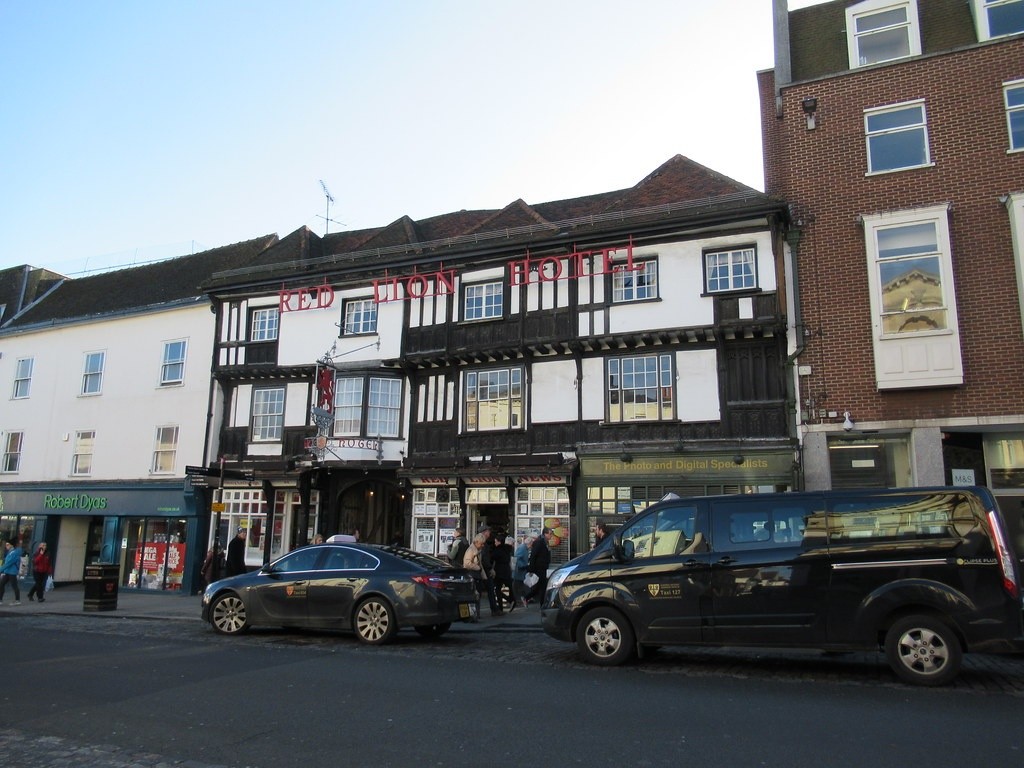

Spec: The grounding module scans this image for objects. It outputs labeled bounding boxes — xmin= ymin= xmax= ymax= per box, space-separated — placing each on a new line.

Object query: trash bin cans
xmin=83 ymin=565 xmax=120 ymax=612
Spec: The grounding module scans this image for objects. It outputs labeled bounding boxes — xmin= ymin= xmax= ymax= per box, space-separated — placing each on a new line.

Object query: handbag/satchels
xmin=524 ymin=572 xmax=540 ymax=587
xmin=45 ymin=574 xmax=54 ymax=593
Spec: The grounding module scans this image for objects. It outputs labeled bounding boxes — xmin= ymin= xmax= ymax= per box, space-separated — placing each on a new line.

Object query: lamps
xmin=801 ymin=98 xmax=817 ymax=130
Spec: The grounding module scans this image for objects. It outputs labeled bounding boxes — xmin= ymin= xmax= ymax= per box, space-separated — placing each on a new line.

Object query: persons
xmin=226 ymin=526 xmax=247 ymax=577
xmin=445 ymin=522 xmax=609 ymax=620
xmin=0 ymin=538 xmax=24 ymax=607
xmin=200 ymin=537 xmax=225 ymax=584
xmin=27 ymin=542 xmax=53 ymax=602
xmin=310 ymin=530 xmax=360 ymax=545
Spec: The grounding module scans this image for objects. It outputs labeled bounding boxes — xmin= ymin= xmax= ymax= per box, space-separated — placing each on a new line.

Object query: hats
xmin=478 ymin=525 xmax=491 ymax=533
xmin=237 ymin=524 xmax=247 ymax=534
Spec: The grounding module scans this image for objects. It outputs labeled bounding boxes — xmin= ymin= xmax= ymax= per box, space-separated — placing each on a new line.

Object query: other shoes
xmin=520 ymin=596 xmax=528 ymax=609
xmin=38 ymin=598 xmax=45 ymax=602
xmin=491 ymin=610 xmax=507 ymax=617
xmin=27 ymin=594 xmax=34 ymax=601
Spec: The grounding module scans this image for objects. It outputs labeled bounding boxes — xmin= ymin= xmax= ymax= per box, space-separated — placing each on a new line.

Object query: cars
xmin=201 ymin=541 xmax=481 ymax=646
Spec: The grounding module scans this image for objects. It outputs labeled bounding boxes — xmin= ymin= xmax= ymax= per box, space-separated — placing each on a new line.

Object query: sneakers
xmin=9 ymin=600 xmax=21 ymax=606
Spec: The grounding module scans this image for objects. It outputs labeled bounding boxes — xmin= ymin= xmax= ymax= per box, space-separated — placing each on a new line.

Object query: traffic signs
xmin=185 ymin=465 xmax=221 ymax=477
xmin=224 ymin=468 xmax=255 ymax=482
xmin=190 ymin=475 xmax=219 ymax=490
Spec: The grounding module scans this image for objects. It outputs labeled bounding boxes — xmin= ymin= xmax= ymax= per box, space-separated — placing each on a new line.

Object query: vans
xmin=542 ymin=485 xmax=1020 ymax=684
